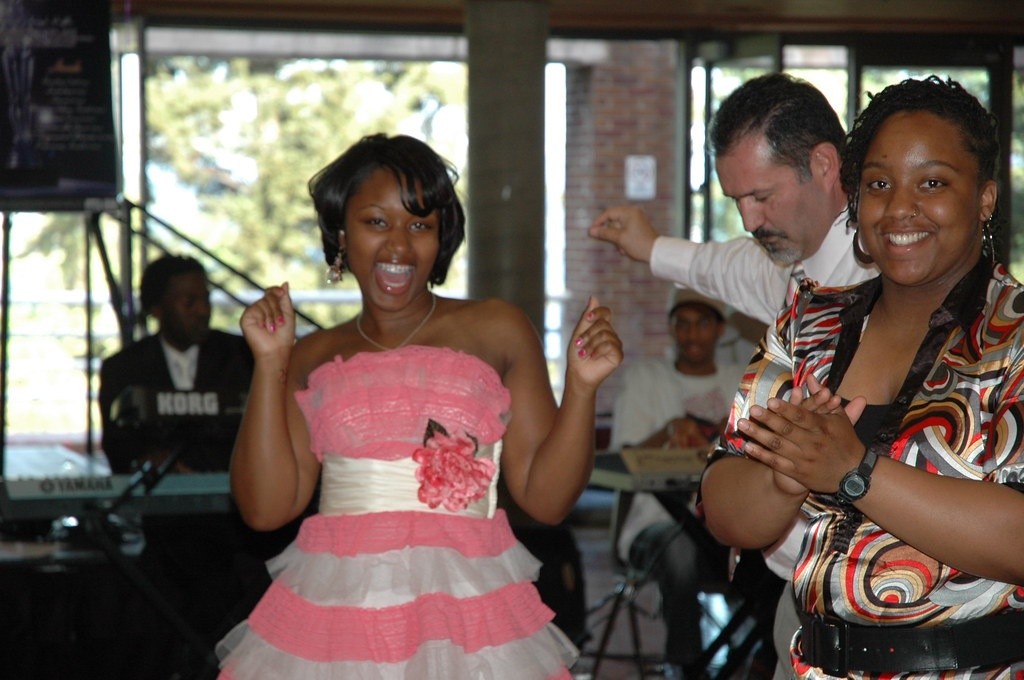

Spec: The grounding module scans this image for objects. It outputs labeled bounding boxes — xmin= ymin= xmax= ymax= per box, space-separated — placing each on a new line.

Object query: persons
xmin=608 ymin=286 xmax=742 ymax=680
xmin=695 ymin=73 xmax=1024 ymax=680
xmin=98 ymin=256 xmax=258 ymax=479
xmin=214 ymin=132 xmax=625 ymax=680
xmin=589 ymin=75 xmax=881 ymax=325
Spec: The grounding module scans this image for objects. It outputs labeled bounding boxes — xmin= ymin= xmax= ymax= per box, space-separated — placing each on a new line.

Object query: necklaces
xmin=356 ymin=292 xmax=436 ymax=352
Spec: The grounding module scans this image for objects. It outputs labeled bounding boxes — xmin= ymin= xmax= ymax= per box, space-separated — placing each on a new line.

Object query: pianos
xmin=0 ymin=468 xmax=232 ymax=529
xmin=586 ymin=448 xmax=708 ymax=493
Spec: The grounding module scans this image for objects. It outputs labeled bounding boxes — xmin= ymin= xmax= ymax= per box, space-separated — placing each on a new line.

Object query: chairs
xmin=608 ymin=495 xmax=679 ymax=680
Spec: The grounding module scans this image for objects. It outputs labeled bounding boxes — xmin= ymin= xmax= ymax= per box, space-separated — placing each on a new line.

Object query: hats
xmin=669 ymin=288 xmax=726 ymax=321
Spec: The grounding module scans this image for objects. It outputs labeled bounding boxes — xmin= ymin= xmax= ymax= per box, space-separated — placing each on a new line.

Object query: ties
xmin=781 ymin=259 xmax=806 ymax=309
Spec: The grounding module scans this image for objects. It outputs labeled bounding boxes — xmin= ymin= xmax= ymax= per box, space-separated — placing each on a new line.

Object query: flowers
xmin=414 ymin=420 xmax=499 ymax=512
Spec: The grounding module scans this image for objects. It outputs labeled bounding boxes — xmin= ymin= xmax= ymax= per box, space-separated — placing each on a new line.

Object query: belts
xmin=799 ymin=612 xmax=1024 ymax=678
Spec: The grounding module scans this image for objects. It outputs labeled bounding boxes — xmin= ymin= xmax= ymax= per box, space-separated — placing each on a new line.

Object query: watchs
xmin=836 ymin=447 xmax=879 ymax=508
